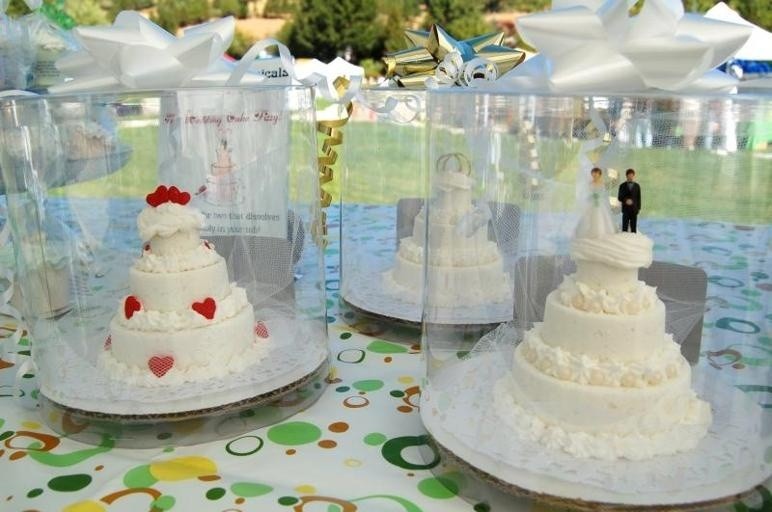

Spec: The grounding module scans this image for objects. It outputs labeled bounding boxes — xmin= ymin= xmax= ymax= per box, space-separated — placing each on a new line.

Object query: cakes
xmin=490 ymin=166 xmax=714 ymax=463
xmin=102 ymin=185 xmax=273 ymax=390
xmin=380 ymin=168 xmax=515 ymax=309
xmin=199 ymin=140 xmax=245 ymax=204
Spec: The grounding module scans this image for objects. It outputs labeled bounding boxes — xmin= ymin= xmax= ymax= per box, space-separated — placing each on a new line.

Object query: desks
xmin=0 ymin=193 xmax=771 ymax=511
